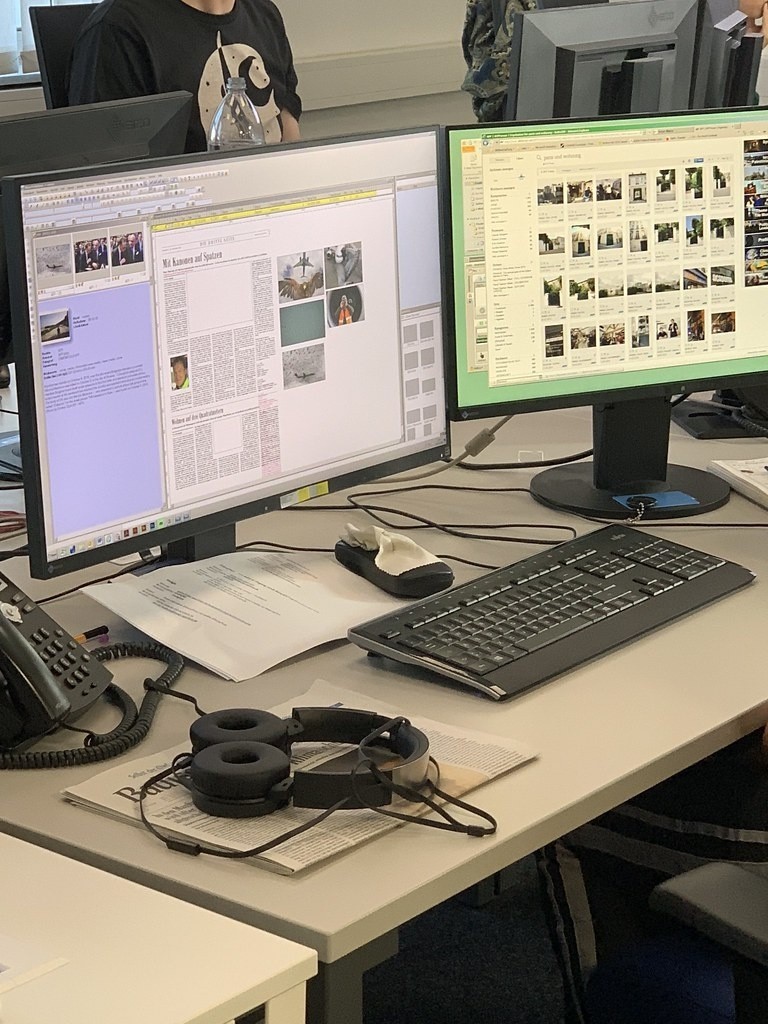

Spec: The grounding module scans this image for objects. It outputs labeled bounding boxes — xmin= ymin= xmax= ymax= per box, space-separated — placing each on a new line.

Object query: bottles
xmin=208 ymin=77 xmax=267 ymax=152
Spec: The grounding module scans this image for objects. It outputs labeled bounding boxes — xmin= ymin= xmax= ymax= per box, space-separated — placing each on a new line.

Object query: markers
xmin=79 ymin=634 xmax=110 ymax=649
xmin=74 ymin=624 xmax=110 ymax=643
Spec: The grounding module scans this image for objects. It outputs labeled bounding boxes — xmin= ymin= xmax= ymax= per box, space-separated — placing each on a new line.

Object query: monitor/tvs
xmin=0 ymin=0 xmax=768 ymax=580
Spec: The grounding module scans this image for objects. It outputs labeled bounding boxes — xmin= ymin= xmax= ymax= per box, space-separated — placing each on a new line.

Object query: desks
xmin=0 ymin=400 xmax=768 ymax=1024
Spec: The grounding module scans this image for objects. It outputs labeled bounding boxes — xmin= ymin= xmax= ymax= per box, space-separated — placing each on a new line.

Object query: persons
xmin=67 ymin=0 xmax=303 ymax=154
xmin=173 ymin=357 xmax=190 ymax=389
xmin=77 ymin=233 xmax=143 ymax=273
xmin=461 ymin=0 xmax=767 ymax=124
xmin=584 ymin=187 xmax=768 ymax=340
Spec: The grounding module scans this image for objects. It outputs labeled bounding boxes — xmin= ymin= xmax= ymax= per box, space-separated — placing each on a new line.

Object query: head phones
xmin=190 ymin=706 xmax=430 ymax=819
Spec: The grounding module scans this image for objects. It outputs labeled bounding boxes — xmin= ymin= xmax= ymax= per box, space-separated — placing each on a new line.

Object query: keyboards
xmin=348 ymin=526 xmax=757 ymax=702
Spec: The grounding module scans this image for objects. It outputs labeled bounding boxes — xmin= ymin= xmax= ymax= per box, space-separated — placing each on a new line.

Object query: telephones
xmin=0 ymin=572 xmax=115 ymax=751
